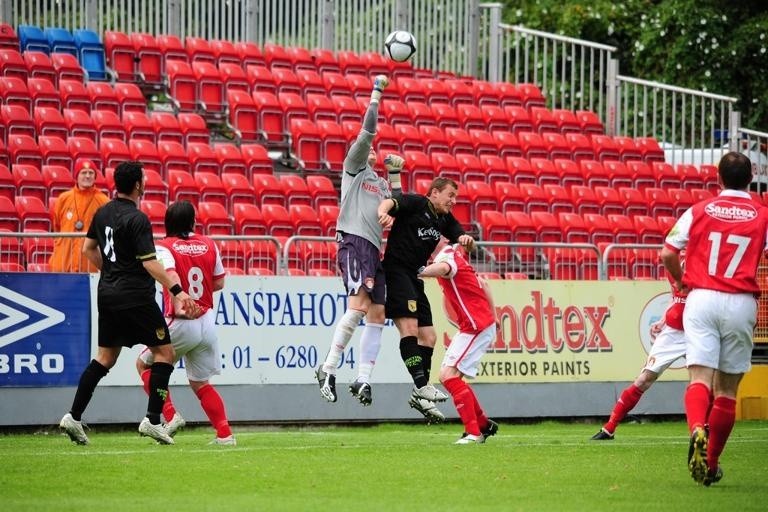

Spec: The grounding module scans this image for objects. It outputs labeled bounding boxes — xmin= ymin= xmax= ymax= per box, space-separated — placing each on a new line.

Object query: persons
xmin=377 ymin=177 xmax=478 ymax=423
xmin=48 ymin=158 xmax=112 ymax=273
xmin=58 ymin=160 xmax=199 ymax=445
xmin=316 ymin=75 xmax=404 ymax=407
xmin=658 ymin=150 xmax=768 ymax=487
xmin=586 ymin=249 xmax=716 ymax=442
xmin=416 ymin=232 xmax=498 ymax=444
xmin=134 ymin=200 xmax=237 ymax=448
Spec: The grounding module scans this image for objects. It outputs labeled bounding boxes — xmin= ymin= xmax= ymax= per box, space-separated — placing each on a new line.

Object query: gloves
xmin=374 ymin=75 xmax=389 ymax=94
xmin=382 ymin=155 xmax=404 ymax=173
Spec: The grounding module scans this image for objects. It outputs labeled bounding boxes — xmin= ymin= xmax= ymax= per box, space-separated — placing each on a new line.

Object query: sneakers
xmin=138 ymin=412 xmax=186 ymax=445
xmin=315 ymin=362 xmax=335 ymax=404
xmin=589 ymin=430 xmax=613 ymax=440
xmin=454 ymin=419 xmax=499 ymax=447
xmin=206 ymin=435 xmax=236 ymax=447
xmin=685 ymin=427 xmax=723 ymax=486
xmin=407 ymin=384 xmax=448 ymax=422
xmin=59 ymin=412 xmax=89 ymax=444
xmin=349 ymin=381 xmax=372 ymax=406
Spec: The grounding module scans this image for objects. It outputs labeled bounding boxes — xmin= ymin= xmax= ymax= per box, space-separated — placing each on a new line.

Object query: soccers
xmin=384 ymin=30 xmax=418 ymax=62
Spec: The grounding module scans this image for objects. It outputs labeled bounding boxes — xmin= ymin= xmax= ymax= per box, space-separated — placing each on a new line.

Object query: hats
xmin=73 ymin=160 xmax=99 ymax=180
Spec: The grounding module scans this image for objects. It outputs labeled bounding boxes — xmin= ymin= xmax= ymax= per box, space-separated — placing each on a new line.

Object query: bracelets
xmin=169 ymin=283 xmax=183 ymax=298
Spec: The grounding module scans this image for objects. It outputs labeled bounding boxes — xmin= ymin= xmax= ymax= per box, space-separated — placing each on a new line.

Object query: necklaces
xmin=72 ymin=188 xmax=97 ymax=230
